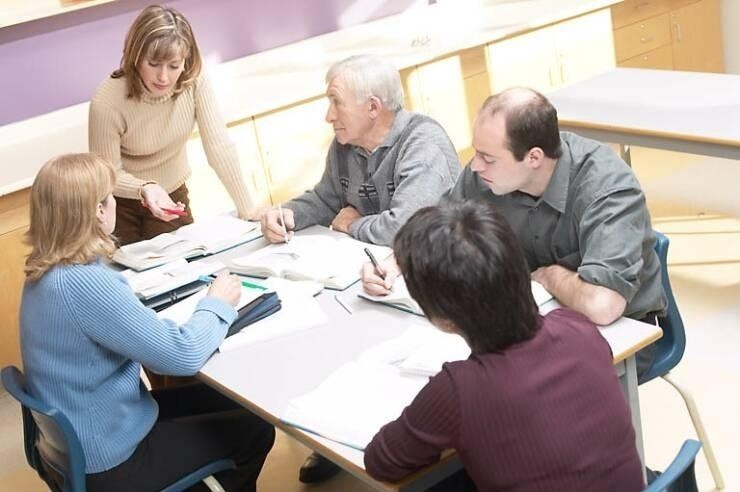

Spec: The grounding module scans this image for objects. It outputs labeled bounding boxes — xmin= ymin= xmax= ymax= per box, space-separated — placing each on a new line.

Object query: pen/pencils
xmin=161 ymin=207 xmax=189 ymax=217
xmin=208 ymin=274 xmax=217 ymax=278
xmin=335 ymin=294 xmax=353 ymax=315
xmin=364 ymin=248 xmax=393 ymax=292
xmin=278 ymin=204 xmax=288 ymax=244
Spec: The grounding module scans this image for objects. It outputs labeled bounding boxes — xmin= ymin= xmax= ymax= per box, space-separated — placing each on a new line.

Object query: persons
xmin=363 ymin=200 xmax=645 ymax=492
xmin=359 ymin=87 xmax=669 ymax=384
xmin=260 ymin=54 xmax=462 ymax=485
xmin=87 ymin=4 xmax=263 ymax=250
xmin=19 ymin=155 xmax=275 ymax=492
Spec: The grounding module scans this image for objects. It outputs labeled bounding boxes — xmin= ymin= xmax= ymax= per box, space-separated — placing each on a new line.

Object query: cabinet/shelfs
xmin=611 ymin=2 xmax=729 ymax=76
xmin=491 ymin=5 xmax=611 ymax=96
xmin=401 ymin=47 xmax=491 ymax=165
xmin=0 ymin=177 xmax=33 ymax=363
xmin=183 ymin=99 xmax=334 ymax=222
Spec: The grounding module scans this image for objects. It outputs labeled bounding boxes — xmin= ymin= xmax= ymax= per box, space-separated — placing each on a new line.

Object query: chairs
xmin=638 ymin=228 xmax=726 ymax=492
xmin=3 ymin=362 xmax=237 ymax=492
xmin=642 ymin=434 xmax=703 ymax=491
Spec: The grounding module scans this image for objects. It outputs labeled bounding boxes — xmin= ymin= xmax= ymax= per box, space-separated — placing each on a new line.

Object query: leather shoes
xmin=299 ymin=450 xmax=341 ymax=481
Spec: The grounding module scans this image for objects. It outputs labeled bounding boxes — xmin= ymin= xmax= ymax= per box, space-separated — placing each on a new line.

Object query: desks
xmin=119 ymin=222 xmax=663 ymax=489
xmin=548 ymin=62 xmax=740 ymax=162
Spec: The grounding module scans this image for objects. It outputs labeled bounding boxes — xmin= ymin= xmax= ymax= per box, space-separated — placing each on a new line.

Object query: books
xmin=358 ymin=254 xmax=554 ymax=318
xmin=112 ymin=215 xmax=375 ymax=338
